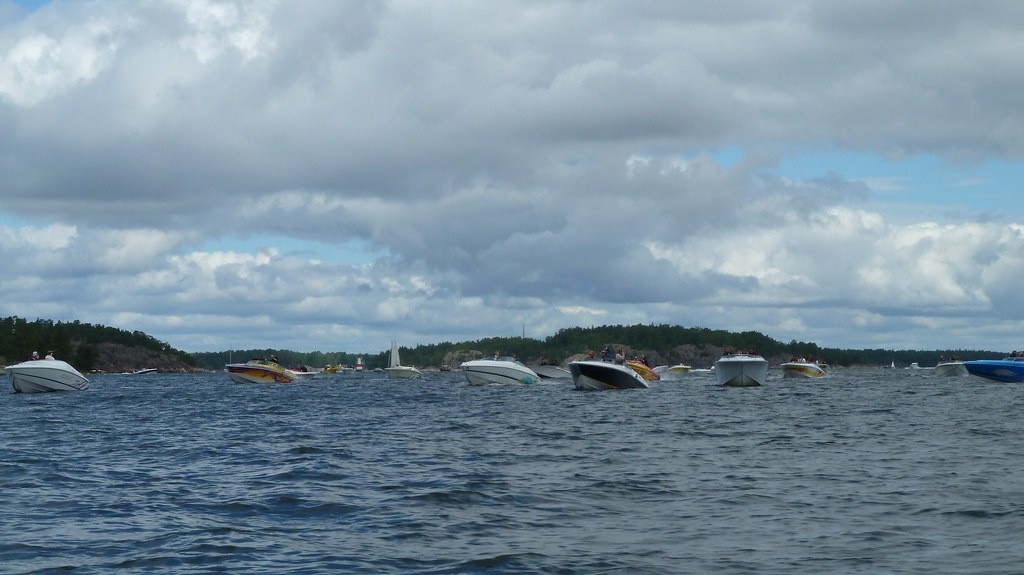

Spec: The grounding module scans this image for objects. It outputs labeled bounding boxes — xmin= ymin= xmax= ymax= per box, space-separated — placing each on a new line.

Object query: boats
xmin=715 ymin=353 xmax=768 ymax=388
xmin=962 ymin=350 xmax=1024 ymax=383
xmin=568 ymin=359 xmax=650 ymax=390
xmin=224 ymin=359 xmax=296 ymax=385
xmin=625 ymin=358 xmax=660 ymax=381
xmin=440 ymin=363 xmax=452 ymax=371
xmin=882 ymin=356 xmax=970 ymax=378
xmin=322 ymin=367 xmax=337 ymax=373
xmin=121 ymin=367 xmax=158 ymax=375
xmin=285 ymin=368 xmax=320 ymax=378
xmin=528 ymin=363 xmax=573 ymax=378
xmin=459 ymin=355 xmax=542 ymax=387
xmin=341 ymin=338 xmax=422 ymax=380
xmin=780 ymin=357 xmax=826 ymax=378
xmin=653 ymin=364 xmax=712 ymax=377
xmin=4 ymin=360 xmax=91 ymax=393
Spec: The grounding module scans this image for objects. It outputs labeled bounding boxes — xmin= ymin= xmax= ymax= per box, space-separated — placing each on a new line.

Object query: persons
xmin=44 ymin=351 xmax=55 ymax=361
xmin=790 ymin=353 xmax=814 ymax=362
xmin=1009 ymin=350 xmax=1017 ymax=357
xmin=722 ymin=345 xmax=757 ymax=356
xmin=585 ymin=345 xmax=625 ymax=365
xmin=270 ymin=354 xmax=280 ymax=365
xmin=29 ymin=351 xmax=40 ymax=361
xmin=492 ymin=350 xmax=517 ymax=362
